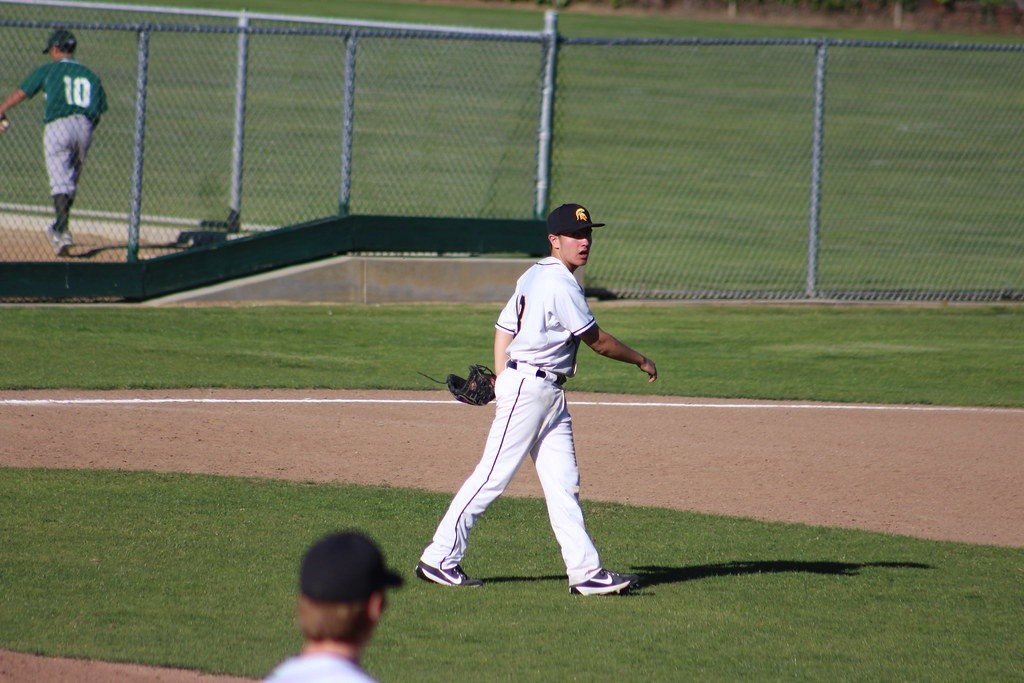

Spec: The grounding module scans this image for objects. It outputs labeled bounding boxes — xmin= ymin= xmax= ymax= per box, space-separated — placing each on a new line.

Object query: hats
xmin=300 ymin=533 xmax=404 ymax=601
xmin=547 ymin=203 xmax=605 ymax=234
xmin=43 ymin=29 xmax=76 ymax=54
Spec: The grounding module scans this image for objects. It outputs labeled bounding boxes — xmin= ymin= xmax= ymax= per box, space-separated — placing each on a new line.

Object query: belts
xmin=507 ymin=360 xmax=566 ymax=385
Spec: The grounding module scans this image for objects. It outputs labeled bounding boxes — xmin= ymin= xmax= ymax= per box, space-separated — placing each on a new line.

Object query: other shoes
xmin=48 ymin=225 xmax=73 ymax=255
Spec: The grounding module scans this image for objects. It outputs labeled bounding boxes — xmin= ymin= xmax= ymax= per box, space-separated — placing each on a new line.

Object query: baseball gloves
xmin=447 ymin=364 xmax=496 ymax=405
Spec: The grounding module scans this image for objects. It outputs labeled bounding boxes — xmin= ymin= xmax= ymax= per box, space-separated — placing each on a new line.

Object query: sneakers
xmin=415 ymin=560 xmax=483 ymax=588
xmin=569 ymin=569 xmax=640 ymax=596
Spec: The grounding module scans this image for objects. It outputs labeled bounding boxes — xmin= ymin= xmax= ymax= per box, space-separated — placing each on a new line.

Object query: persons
xmin=261 ymin=530 xmax=403 ymax=683
xmin=415 ymin=204 xmax=658 ymax=596
xmin=0 ymin=31 xmax=108 ymax=256
